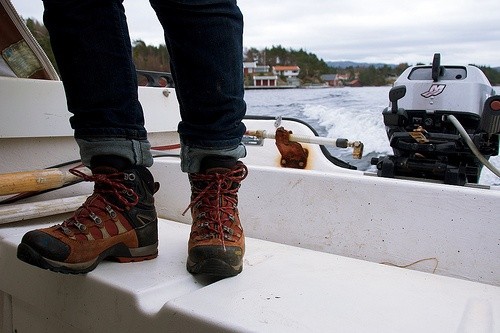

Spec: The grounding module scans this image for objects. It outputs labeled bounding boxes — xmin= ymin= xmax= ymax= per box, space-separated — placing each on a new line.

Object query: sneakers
xmin=17 ymin=154 xmax=160 ymax=275
xmin=182 ymin=154 xmax=248 ymax=275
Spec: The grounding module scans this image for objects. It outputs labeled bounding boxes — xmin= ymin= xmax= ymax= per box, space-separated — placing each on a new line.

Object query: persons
xmin=17 ymin=0 xmax=248 ymax=277
